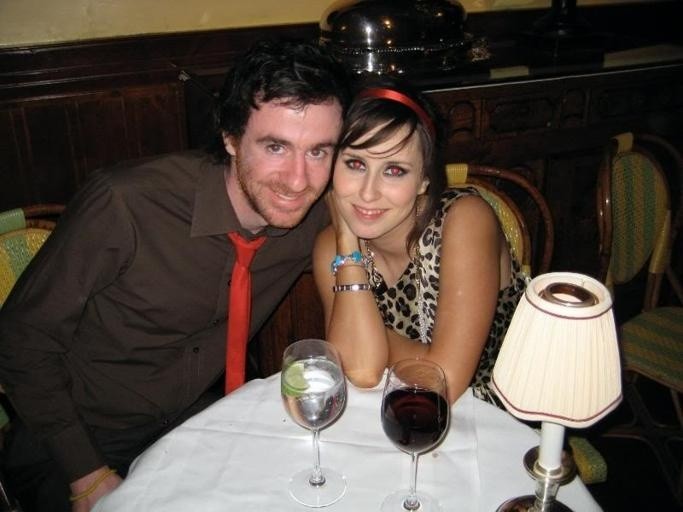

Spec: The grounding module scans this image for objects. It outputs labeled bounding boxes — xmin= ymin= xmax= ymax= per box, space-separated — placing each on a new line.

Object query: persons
xmin=312 ymin=84 xmax=532 ymax=412
xmin=0 ymin=44 xmax=354 ymax=512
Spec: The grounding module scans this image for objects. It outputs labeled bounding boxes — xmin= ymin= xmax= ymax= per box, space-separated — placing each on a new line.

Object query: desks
xmin=90 ymin=356 xmax=604 ymax=512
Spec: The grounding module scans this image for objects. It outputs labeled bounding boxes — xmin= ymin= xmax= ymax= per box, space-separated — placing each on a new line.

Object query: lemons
xmin=281 ymin=361 xmax=311 ymax=394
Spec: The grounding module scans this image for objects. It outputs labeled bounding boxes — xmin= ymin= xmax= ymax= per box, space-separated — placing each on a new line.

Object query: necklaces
xmin=365 ymin=202 xmax=427 ymax=344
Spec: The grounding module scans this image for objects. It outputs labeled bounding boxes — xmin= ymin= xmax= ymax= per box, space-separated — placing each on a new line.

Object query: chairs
xmin=0 ymin=202 xmax=81 ymax=512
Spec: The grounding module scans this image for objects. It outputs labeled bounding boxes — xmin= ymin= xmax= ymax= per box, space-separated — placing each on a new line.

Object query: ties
xmin=225 ymin=231 xmax=267 ymax=393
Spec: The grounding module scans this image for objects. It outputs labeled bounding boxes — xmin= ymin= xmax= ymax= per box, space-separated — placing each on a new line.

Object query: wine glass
xmin=280 ymin=338 xmax=347 ymax=508
xmin=379 ymin=358 xmax=448 ymax=512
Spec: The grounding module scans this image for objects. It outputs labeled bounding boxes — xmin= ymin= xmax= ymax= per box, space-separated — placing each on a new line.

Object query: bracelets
xmin=332 ymin=284 xmax=371 ymax=291
xmin=70 ymin=469 xmax=117 ymax=502
xmin=332 ymin=251 xmax=364 ymax=276
